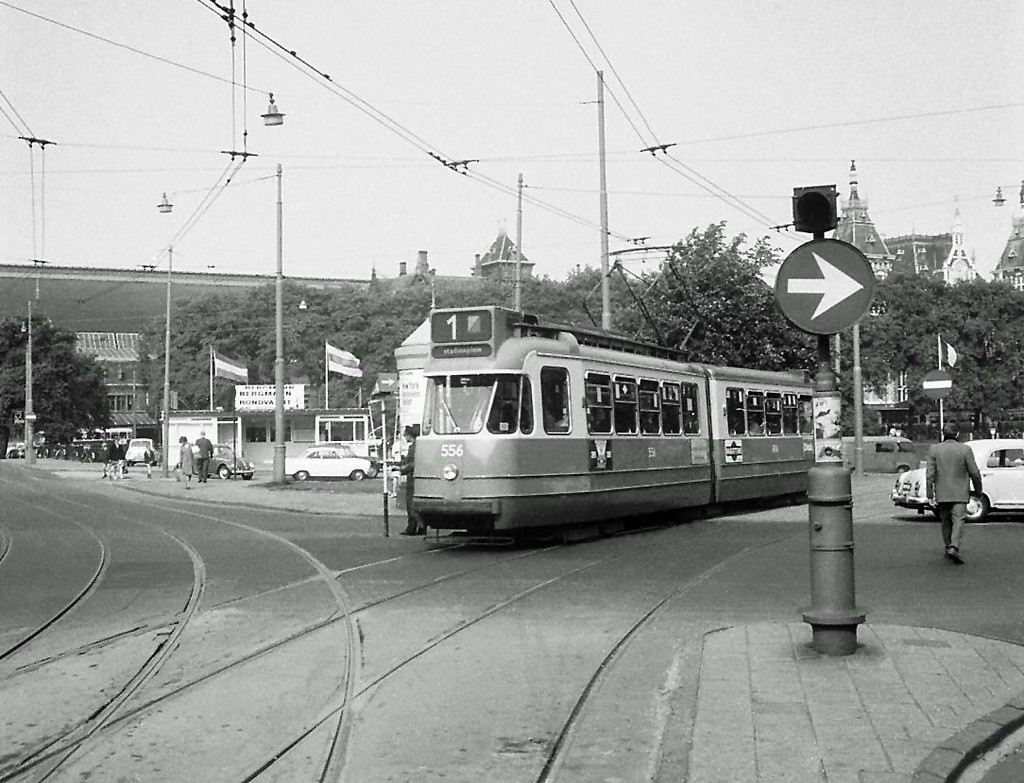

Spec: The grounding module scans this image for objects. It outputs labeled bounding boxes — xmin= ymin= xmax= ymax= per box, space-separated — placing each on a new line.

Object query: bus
xmin=409 ymin=245 xmax=815 ymax=549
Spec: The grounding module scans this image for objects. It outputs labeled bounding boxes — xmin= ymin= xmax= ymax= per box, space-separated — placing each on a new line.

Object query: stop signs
xmin=921 ymin=370 xmax=954 ymax=400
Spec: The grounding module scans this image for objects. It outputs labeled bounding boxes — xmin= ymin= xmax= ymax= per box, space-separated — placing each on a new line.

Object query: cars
xmin=124 ymin=437 xmax=162 ymax=467
xmin=173 ymin=442 xmax=256 ymax=481
xmin=285 ymin=443 xmax=373 ymax=482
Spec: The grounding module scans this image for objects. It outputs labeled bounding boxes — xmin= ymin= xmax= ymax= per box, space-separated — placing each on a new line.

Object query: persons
xmin=196 ymin=432 xmax=215 ymax=483
xmin=925 ymin=423 xmax=982 ymax=564
xmin=101 ymin=436 xmax=156 ymax=480
xmin=178 ymin=436 xmax=197 ymax=489
xmin=390 ymin=426 xmax=428 ymax=536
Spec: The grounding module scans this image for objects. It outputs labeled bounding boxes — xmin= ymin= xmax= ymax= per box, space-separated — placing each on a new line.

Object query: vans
xmin=888 ymin=439 xmax=1024 ymax=523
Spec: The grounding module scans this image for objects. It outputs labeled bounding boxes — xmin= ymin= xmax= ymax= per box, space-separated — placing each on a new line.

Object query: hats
xmin=179 ymin=436 xmax=187 ymax=443
xmin=402 ymin=425 xmax=419 ymax=435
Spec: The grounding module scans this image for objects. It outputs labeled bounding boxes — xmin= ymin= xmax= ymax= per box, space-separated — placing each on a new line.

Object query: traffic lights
xmin=792 ymin=184 xmax=838 ymax=233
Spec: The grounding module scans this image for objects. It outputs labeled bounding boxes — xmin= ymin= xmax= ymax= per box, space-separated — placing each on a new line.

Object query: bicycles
xmin=7 ymin=436 xmax=95 ymax=463
xmin=107 ymin=460 xmax=125 ymax=481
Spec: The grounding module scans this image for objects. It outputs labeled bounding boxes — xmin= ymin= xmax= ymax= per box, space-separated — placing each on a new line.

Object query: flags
xmin=940 ymin=340 xmax=961 ymax=372
xmin=213 ymin=348 xmax=248 ymax=384
xmin=327 ymin=344 xmax=363 ymax=377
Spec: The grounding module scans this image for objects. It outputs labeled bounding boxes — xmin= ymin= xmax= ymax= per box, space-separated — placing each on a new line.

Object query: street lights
xmin=19 ymin=300 xmax=55 ymax=464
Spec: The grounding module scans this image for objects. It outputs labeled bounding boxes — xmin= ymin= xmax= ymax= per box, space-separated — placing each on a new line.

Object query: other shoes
xmin=399 ymin=528 xmax=417 ymax=535
xmin=101 ymin=474 xmax=108 ymax=479
xmin=417 ymin=526 xmax=427 ymax=535
xmin=943 ymin=548 xmax=964 ymax=564
